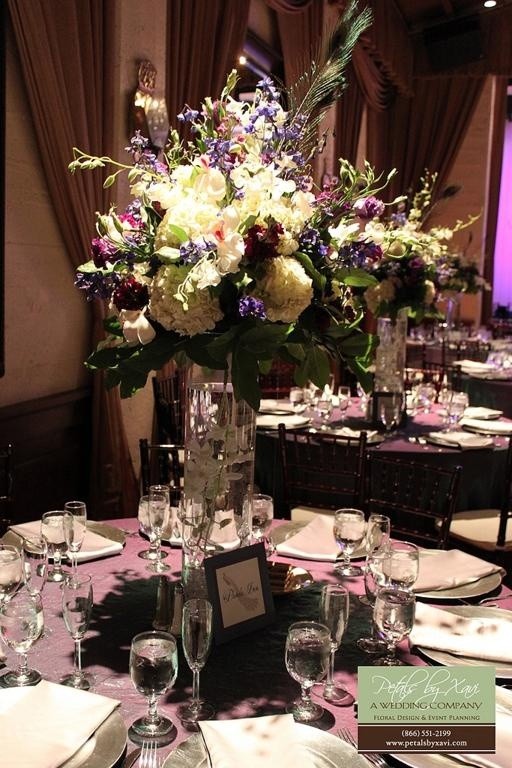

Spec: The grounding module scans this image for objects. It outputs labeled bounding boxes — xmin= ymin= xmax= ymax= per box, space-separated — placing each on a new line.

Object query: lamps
xmin=127 ymin=60 xmax=171 ymax=162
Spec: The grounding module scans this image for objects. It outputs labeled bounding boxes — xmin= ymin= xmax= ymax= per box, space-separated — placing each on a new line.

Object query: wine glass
xmin=0 ymin=590 xmax=46 ymax=689
xmin=136 ymin=482 xmax=177 ymax=573
xmin=287 ymin=373 xmax=355 ymax=431
xmin=127 ymin=631 xmax=180 ymax=747
xmin=282 ymin=620 xmax=334 ymax=723
xmin=1 ymin=501 xmax=88 ymax=602
xmin=175 ymin=597 xmax=217 ymax=724
xmin=312 ymin=582 xmax=352 ymax=700
xmin=332 ymin=505 xmax=418 ymax=672
xmin=58 ymin=573 xmax=96 ymax=690
xmin=246 ymin=493 xmax=275 ymax=558
xmin=415 ymin=382 xmax=466 ymax=435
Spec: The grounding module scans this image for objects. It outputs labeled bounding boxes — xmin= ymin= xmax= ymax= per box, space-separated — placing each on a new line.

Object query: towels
xmin=1 ymin=680 xmax=122 ymax=767
xmin=197 ymin=713 xmax=314 ymax=768
xmin=1 ymin=516 xmax=512 ymax=766
xmin=368 ymin=546 xmax=504 ymax=600
xmin=404 ymin=601 xmax=511 ymax=663
xmin=275 ymin=516 xmax=375 ymax=561
xmin=9 ymin=514 xmax=122 ymax=568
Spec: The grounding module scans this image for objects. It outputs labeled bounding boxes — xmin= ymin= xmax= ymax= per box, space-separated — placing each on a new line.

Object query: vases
xmin=181 ymin=348 xmax=257 ymax=600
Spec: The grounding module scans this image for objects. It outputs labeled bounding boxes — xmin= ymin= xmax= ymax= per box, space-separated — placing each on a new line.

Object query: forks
xmin=336 ymin=725 xmax=390 ymax=767
xmin=138 ymin=740 xmax=159 ymax=768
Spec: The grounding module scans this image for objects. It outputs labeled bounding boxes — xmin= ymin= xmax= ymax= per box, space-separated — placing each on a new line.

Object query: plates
xmin=373 ymin=547 xmax=503 ymax=601
xmin=254 ymin=398 xmax=386 ymax=447
xmin=161 ymin=712 xmax=373 ymax=767
xmin=417 ymin=404 xmax=511 ymax=449
xmin=9 ymin=516 xmax=127 ymax=564
xmin=58 ymin=698 xmax=130 ymax=768
xmin=265 ymin=515 xmax=383 ymax=562
xmin=411 ymin=604 xmax=512 ymax=680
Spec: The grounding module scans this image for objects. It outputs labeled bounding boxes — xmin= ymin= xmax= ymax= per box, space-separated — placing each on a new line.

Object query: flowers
xmin=68 ymin=0 xmax=484 ymax=414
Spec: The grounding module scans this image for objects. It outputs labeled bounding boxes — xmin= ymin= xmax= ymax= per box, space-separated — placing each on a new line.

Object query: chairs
xmin=139 ymin=336 xmax=512 ymax=550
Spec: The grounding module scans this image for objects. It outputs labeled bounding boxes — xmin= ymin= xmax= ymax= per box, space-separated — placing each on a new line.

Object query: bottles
xmin=178 ymin=345 xmax=263 ymax=608
